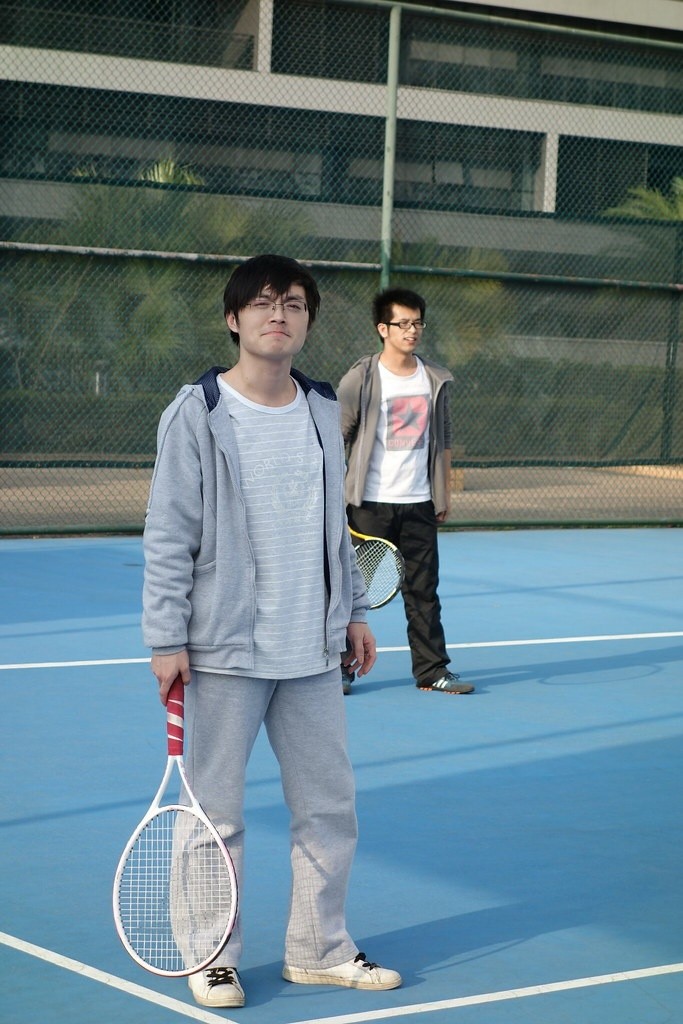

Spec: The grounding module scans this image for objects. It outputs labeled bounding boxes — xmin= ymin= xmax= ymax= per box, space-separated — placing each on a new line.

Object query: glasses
xmin=245 ymin=300 xmax=308 ymax=315
xmin=385 ymin=319 xmax=427 ymax=329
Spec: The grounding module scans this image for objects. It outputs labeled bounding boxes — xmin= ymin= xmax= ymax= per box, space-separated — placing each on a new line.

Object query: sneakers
xmin=342 ymin=673 xmax=351 ymax=694
xmin=282 ymin=952 xmax=402 ymax=990
xmin=188 ymin=965 xmax=245 ymax=1006
xmin=420 ymin=671 xmax=475 ymax=694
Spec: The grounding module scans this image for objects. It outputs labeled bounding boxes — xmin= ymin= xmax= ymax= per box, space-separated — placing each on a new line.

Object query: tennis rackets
xmin=112 ymin=677 xmax=240 ymax=979
xmin=346 ymin=526 xmax=407 ymax=610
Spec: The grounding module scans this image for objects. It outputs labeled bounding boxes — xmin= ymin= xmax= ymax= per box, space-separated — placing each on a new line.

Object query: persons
xmin=141 ymin=254 xmax=403 ymax=1008
xmin=333 ymin=290 xmax=475 ymax=695
